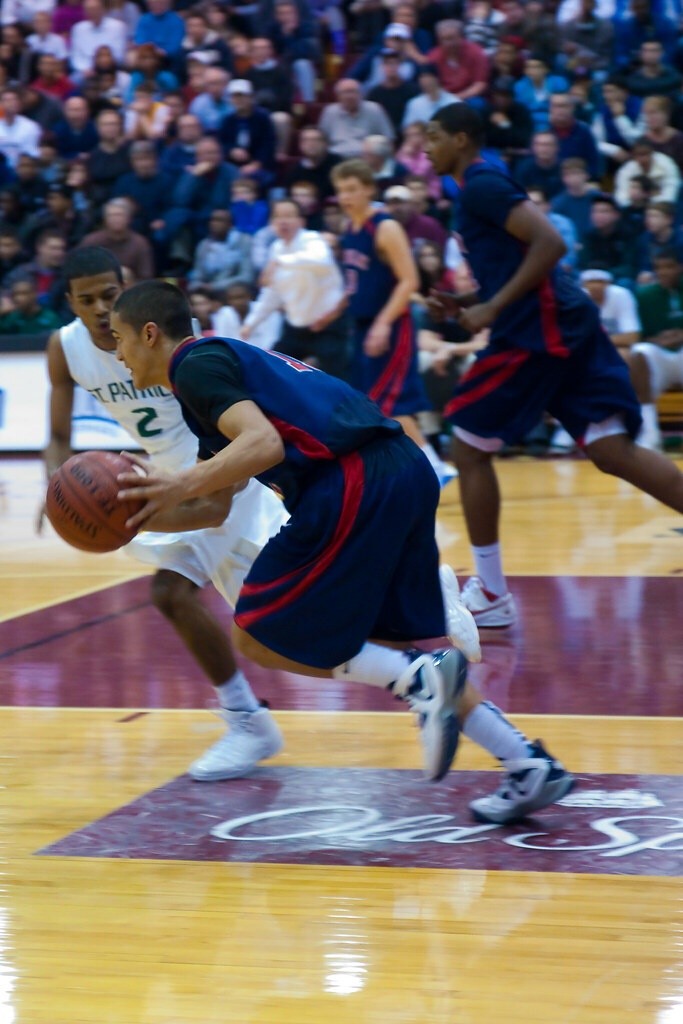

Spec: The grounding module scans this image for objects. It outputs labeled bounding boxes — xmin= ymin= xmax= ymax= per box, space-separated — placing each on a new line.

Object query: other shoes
xmin=497 ymin=438 xmax=548 ymax=459
xmin=549 ymin=426 xmax=575 ymax=454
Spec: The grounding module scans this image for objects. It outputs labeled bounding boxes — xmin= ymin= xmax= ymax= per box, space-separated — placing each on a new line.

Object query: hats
xmin=226 ymin=79 xmax=251 ymax=95
xmin=386 ymin=22 xmax=411 ymax=39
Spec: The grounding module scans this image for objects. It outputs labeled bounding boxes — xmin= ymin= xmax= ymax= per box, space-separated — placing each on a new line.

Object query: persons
xmin=36 ymin=244 xmax=469 ymax=783
xmin=0 ymin=1 xmax=683 ymax=451
xmin=109 ymin=280 xmax=577 ymax=826
xmin=330 ymin=159 xmax=459 ymax=488
xmin=422 ymin=102 xmax=683 ymax=630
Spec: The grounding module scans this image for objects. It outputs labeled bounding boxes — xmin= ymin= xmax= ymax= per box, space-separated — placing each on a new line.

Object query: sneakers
xmin=384 ymin=645 xmax=468 ymax=783
xmin=469 ymin=738 xmax=574 ymax=823
xmin=191 ymin=698 xmax=283 ymax=780
xmin=459 ymin=577 xmax=516 ymax=626
xmin=439 ymin=564 xmax=481 ymax=663
xmin=437 ymin=462 xmax=458 ymax=489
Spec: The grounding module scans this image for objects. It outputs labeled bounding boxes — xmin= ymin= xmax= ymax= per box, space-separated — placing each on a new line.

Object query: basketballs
xmin=45 ymin=451 xmax=149 ymax=553
